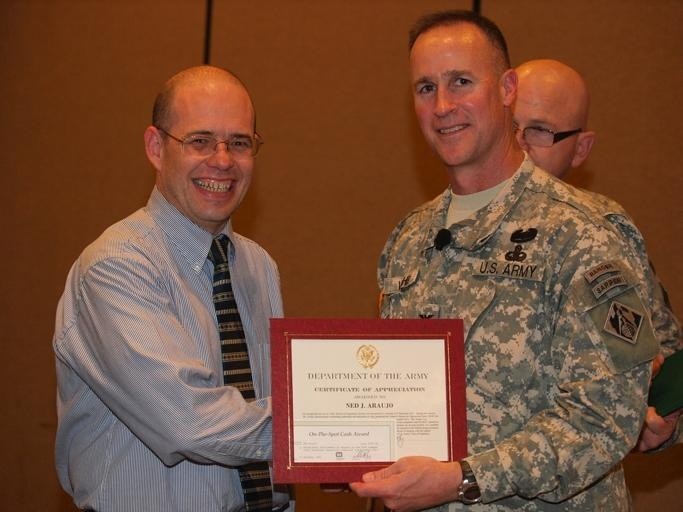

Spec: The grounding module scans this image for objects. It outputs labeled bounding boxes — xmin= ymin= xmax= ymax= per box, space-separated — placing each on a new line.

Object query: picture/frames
xmin=270 ymin=317 xmax=467 ymax=484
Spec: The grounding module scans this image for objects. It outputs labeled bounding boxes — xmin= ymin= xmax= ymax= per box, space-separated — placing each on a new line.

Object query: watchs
xmin=457 ymin=459 xmax=482 ymax=505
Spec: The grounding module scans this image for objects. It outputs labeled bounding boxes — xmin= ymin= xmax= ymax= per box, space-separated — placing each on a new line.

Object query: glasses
xmin=155 ymin=126 xmax=262 ymax=157
xmin=515 ymin=124 xmax=583 ymax=149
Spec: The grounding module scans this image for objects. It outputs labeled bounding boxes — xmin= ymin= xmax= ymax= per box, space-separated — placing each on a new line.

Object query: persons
xmin=505 ymin=59 xmax=683 ymax=456
xmin=49 ymin=64 xmax=300 ymax=511
xmin=349 ymin=9 xmax=661 ymax=512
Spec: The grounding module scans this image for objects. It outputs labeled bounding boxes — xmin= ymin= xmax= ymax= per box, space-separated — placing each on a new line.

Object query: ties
xmin=207 ymin=235 xmax=272 ymax=510
